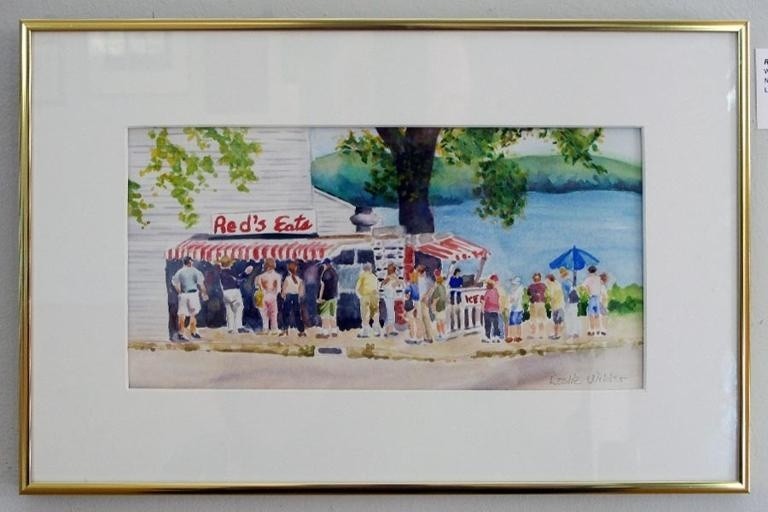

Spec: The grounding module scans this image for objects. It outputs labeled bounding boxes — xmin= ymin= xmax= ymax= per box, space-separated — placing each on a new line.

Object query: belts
xmin=180 ymin=289 xmax=198 ymax=294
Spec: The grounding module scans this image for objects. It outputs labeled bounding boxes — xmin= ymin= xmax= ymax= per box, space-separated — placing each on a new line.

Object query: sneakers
xmin=527 ymin=333 xmax=579 ymax=340
xmin=238 ymin=327 xmax=250 ymax=334
xmin=481 ymin=336 xmax=523 ymax=344
xmin=587 ymin=330 xmax=607 ymax=337
xmin=404 ymin=332 xmax=447 ymax=345
xmin=190 ymin=332 xmax=201 ymax=339
xmin=357 ymin=331 xmax=399 ymax=338
xmin=332 ymin=333 xmax=338 ymax=337
xmin=256 ymin=328 xmax=307 ymax=337
xmin=177 ymin=334 xmax=189 ymax=342
xmin=316 ymin=333 xmax=330 ymax=339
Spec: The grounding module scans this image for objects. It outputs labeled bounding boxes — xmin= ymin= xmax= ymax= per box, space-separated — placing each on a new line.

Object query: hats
xmin=321 ymin=258 xmax=331 ymax=265
xmin=220 ymin=255 xmax=234 ymax=268
xmin=182 ymin=256 xmax=195 ymax=265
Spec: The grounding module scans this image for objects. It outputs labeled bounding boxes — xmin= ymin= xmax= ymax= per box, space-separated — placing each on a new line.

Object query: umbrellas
xmin=549 ymin=244 xmax=602 ymax=285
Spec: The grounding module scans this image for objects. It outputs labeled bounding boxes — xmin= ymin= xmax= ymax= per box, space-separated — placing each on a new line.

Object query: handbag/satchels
xmin=252 ymin=277 xmax=264 ymax=308
xmin=404 ymin=285 xmax=414 ymax=312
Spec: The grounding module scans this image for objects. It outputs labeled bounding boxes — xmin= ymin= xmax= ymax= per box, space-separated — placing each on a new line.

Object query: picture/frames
xmin=17 ymin=17 xmax=750 ymax=494
